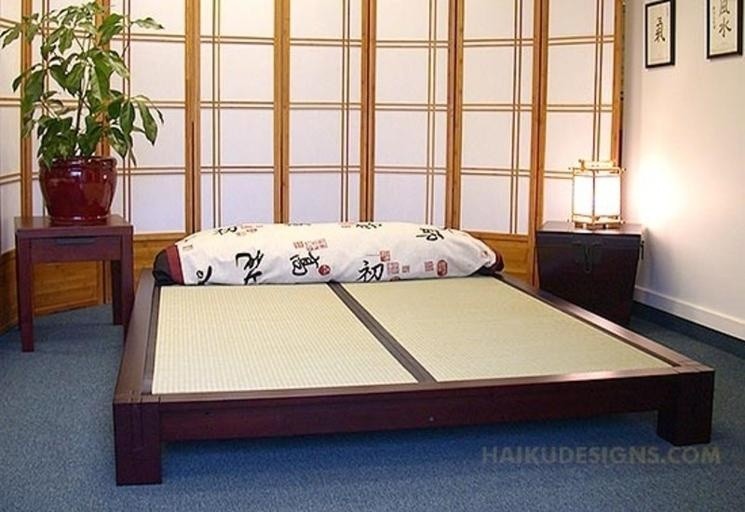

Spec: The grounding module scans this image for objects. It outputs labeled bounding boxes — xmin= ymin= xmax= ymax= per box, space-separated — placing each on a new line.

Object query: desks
xmin=13 ymin=213 xmax=136 ymax=351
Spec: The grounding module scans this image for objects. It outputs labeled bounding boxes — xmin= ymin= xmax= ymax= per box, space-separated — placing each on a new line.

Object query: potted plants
xmin=0 ymin=0 xmax=167 ymax=223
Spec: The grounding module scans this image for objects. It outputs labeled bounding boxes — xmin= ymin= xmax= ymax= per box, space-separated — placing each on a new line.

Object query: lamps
xmin=570 ymin=158 xmax=630 ymax=230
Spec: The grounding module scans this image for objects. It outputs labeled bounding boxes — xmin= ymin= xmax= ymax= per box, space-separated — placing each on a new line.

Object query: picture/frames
xmin=707 ymin=0 xmax=743 ymax=58
xmin=644 ymin=2 xmax=676 ymax=68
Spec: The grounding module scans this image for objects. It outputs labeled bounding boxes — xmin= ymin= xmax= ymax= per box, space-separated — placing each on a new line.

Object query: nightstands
xmin=535 ymin=221 xmax=644 ymax=328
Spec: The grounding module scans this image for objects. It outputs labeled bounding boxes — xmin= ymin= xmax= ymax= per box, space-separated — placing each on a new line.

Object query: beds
xmin=112 ymin=221 xmax=716 ymax=484
